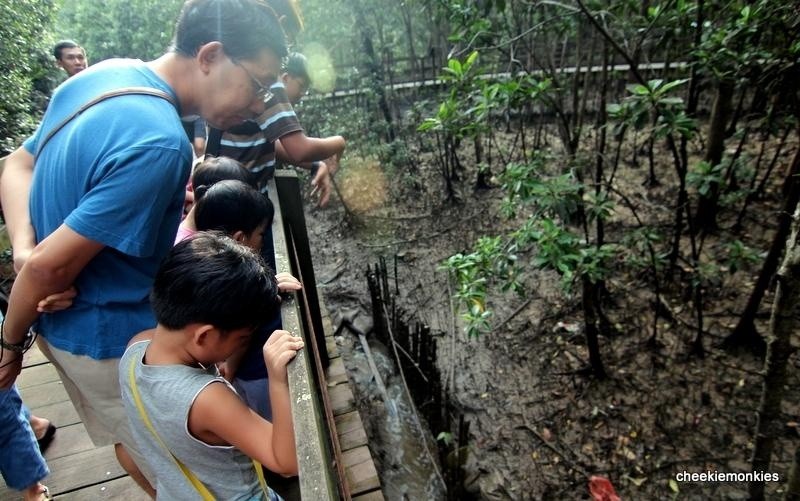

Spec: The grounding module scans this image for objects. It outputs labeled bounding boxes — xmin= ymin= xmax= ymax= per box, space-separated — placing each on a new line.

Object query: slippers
xmin=36 ymin=423 xmax=57 ymax=453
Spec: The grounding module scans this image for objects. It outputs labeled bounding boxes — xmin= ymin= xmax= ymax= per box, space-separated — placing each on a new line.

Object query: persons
xmin=0 ymin=0 xmax=347 ymax=501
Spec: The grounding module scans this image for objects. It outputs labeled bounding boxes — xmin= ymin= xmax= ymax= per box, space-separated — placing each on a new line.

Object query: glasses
xmin=238 ymin=61 xmax=275 ymax=104
